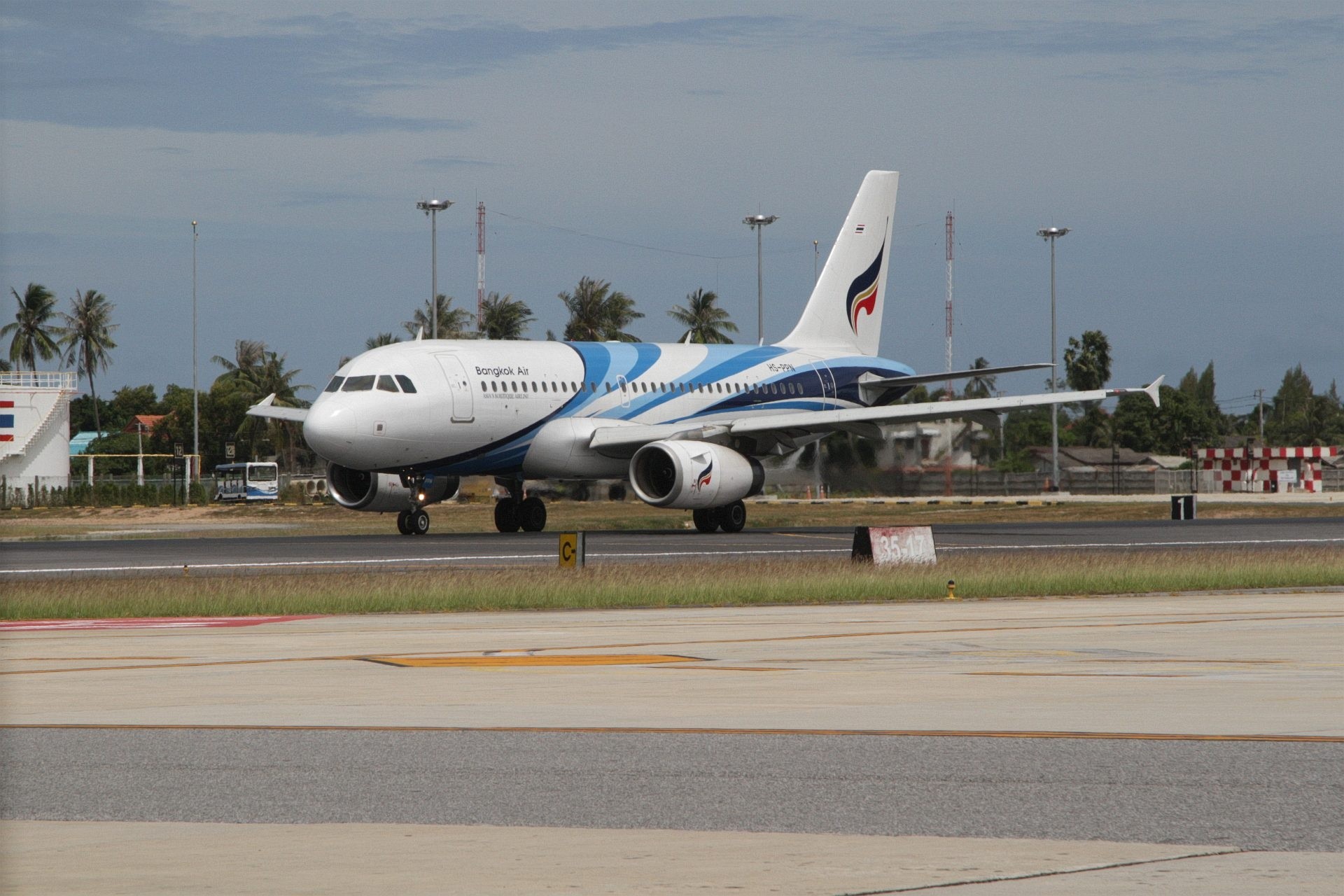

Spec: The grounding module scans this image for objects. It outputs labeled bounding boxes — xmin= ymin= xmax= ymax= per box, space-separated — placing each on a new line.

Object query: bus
xmin=212 ymin=461 xmax=279 ymax=504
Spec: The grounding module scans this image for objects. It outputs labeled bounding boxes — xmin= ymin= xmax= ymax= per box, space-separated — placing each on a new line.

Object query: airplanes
xmin=243 ymin=171 xmax=1171 ymax=535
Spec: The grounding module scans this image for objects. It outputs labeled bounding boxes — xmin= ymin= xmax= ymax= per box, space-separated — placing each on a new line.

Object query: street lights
xmin=415 ymin=196 xmax=456 ymax=340
xmin=1033 ymin=225 xmax=1075 ymax=492
xmin=740 ymin=213 xmax=780 ymax=343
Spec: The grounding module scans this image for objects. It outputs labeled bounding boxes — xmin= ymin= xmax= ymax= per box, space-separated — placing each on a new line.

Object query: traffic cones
xmin=806 ymin=484 xmax=812 ymax=500
xmin=820 ymin=484 xmax=826 ymax=500
